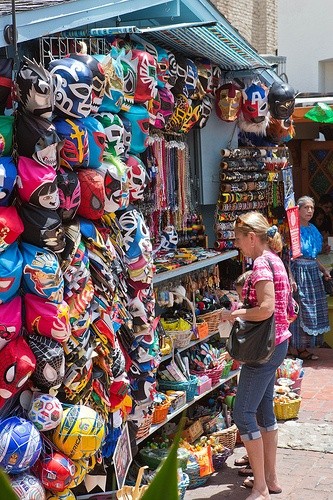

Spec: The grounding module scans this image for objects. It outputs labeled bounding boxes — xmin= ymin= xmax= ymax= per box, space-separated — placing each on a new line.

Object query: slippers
xmin=242 ymin=478 xmax=282 ymax=494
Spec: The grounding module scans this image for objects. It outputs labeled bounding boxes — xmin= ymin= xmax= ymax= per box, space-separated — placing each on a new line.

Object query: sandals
xmin=234 ymin=455 xmax=249 ymax=466
xmin=286 ymin=353 xmax=296 ymax=360
xmin=298 ymin=350 xmax=319 ymax=360
xmin=238 ymin=465 xmax=254 ymax=477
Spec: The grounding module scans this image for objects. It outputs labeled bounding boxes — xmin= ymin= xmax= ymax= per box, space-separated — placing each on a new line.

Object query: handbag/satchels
xmin=225 ymin=258 xmax=276 ymax=364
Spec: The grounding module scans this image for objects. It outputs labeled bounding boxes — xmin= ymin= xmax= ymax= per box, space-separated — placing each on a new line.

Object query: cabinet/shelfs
xmin=129 ymin=250 xmax=240 ymax=444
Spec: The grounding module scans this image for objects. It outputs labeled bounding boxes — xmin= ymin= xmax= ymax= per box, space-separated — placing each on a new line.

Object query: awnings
xmin=0 ymin=0 xmax=283 ymax=87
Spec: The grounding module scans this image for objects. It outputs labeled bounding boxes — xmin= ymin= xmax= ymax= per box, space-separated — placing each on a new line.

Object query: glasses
xmin=236 ymin=217 xmax=254 ymax=231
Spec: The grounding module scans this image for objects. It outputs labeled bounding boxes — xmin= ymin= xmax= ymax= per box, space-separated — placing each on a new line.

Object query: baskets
xmin=136 ymin=358 xmax=240 ymax=440
xmin=159 ymin=292 xmax=196 ymax=347
xmin=208 ymin=423 xmax=238 ymax=450
xmin=138 ymin=448 xmax=210 ymax=489
xmin=273 ymin=396 xmax=302 ymax=420
xmin=141 ymin=471 xmax=190 ymax=500
xmin=194 ymin=306 xmax=225 ymax=332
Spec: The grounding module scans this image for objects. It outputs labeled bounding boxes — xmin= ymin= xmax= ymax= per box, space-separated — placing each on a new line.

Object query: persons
xmin=232 ymin=211 xmax=296 ymax=500
xmin=284 ymin=196 xmax=333 ymax=359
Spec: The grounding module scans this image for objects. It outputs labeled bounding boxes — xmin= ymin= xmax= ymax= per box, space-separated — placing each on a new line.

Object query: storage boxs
xmin=169 ymin=420 xmax=203 ymax=444
